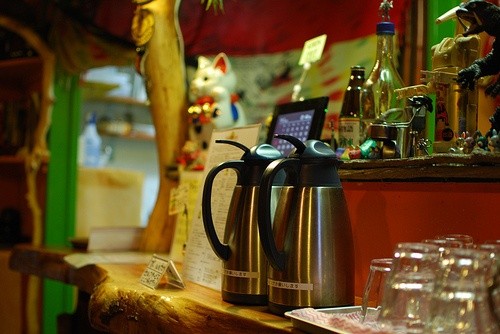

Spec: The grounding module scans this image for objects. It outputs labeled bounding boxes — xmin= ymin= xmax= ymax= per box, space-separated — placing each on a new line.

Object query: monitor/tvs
xmin=265 ymin=95 xmax=330 ymax=158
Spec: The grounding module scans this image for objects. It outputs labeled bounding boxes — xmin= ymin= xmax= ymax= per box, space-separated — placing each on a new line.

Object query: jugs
xmin=260 ymin=134 xmax=350 ymax=317
xmin=203 ymin=139 xmax=288 ymax=306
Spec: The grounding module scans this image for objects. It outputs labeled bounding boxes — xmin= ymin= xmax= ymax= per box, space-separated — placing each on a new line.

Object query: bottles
xmin=338 ymin=65 xmax=367 ymax=161
xmin=429 ymin=34 xmax=479 ymax=151
xmin=75 ymin=112 xmax=101 ymax=170
xmin=359 ymin=22 xmax=406 ymax=159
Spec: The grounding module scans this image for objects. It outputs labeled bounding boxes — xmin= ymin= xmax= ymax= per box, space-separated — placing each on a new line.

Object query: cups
xmin=362 ymin=234 xmax=500 ymax=333
xmin=369 ymin=121 xmax=415 ymax=159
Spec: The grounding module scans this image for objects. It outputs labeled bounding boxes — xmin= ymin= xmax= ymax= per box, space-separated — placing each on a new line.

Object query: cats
xmin=186 ymin=51 xmax=246 ymax=172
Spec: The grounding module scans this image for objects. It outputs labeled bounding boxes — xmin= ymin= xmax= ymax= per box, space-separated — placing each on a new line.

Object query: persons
xmin=79 ymin=111 xmax=152 ymax=166
